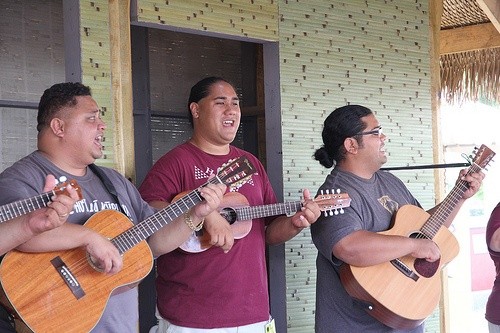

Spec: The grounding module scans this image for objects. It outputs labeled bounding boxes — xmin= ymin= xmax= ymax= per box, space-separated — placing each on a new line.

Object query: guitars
xmin=171 ymin=189 xmax=352 ymax=254
xmin=0 ymin=176 xmax=84 ymax=223
xmin=0 ymin=156 xmax=257 ymax=332
xmin=340 ymin=143 xmax=496 ymax=330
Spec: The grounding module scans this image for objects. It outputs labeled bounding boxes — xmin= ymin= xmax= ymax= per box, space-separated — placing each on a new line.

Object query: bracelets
xmin=185 ymin=205 xmax=207 ymax=233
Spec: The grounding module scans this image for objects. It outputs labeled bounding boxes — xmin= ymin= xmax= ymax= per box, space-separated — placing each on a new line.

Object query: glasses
xmin=355 ymin=126 xmax=382 ymax=137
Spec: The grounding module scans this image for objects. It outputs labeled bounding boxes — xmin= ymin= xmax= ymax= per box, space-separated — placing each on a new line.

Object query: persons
xmin=137 ymin=76 xmax=321 ymax=332
xmin=0 ymin=173 xmax=80 ymax=255
xmin=310 ymin=104 xmax=485 ymax=333
xmin=0 ymin=80 xmax=227 ymax=333
xmin=483 ymin=201 xmax=500 ymax=333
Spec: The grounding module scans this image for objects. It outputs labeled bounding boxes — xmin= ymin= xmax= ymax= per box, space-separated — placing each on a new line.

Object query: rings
xmin=59 ymin=211 xmax=69 ymax=218
